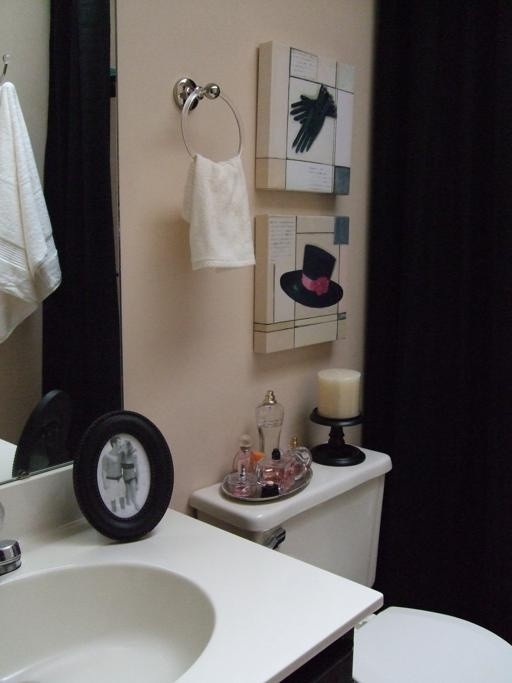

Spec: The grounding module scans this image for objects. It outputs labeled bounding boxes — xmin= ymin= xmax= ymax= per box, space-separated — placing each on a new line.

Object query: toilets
xmin=189 ymin=445 xmax=512 ymax=682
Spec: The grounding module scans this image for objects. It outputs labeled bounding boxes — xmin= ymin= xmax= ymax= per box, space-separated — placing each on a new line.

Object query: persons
xmin=102 ymin=436 xmax=141 ymax=512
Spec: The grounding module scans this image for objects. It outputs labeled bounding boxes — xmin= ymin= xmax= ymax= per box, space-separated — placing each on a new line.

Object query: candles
xmin=306 ymin=369 xmax=365 ymax=422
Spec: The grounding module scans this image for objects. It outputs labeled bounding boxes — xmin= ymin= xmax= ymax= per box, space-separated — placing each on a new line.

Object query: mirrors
xmin=1 ymin=1 xmax=125 ymax=488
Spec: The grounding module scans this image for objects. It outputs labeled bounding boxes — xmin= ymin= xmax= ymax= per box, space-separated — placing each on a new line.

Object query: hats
xmin=280 ymin=244 xmax=344 ymax=308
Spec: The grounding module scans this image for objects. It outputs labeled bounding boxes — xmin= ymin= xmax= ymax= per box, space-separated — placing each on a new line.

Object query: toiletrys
xmin=223 ymin=390 xmax=312 ymax=498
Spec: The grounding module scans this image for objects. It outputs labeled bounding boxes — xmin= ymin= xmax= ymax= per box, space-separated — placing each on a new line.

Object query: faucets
xmin=0 ymin=502 xmax=22 ymax=575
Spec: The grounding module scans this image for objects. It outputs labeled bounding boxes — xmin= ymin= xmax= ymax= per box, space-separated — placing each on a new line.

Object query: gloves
xmin=290 ymin=84 xmax=337 ymax=154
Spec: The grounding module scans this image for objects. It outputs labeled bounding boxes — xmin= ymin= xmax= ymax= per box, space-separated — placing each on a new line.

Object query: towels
xmin=1 ymin=83 xmax=63 ymax=346
xmin=182 ymin=148 xmax=258 ymax=271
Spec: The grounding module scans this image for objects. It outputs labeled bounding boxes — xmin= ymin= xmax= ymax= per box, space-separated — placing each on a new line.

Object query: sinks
xmin=0 ymin=553 xmax=218 ymax=683
